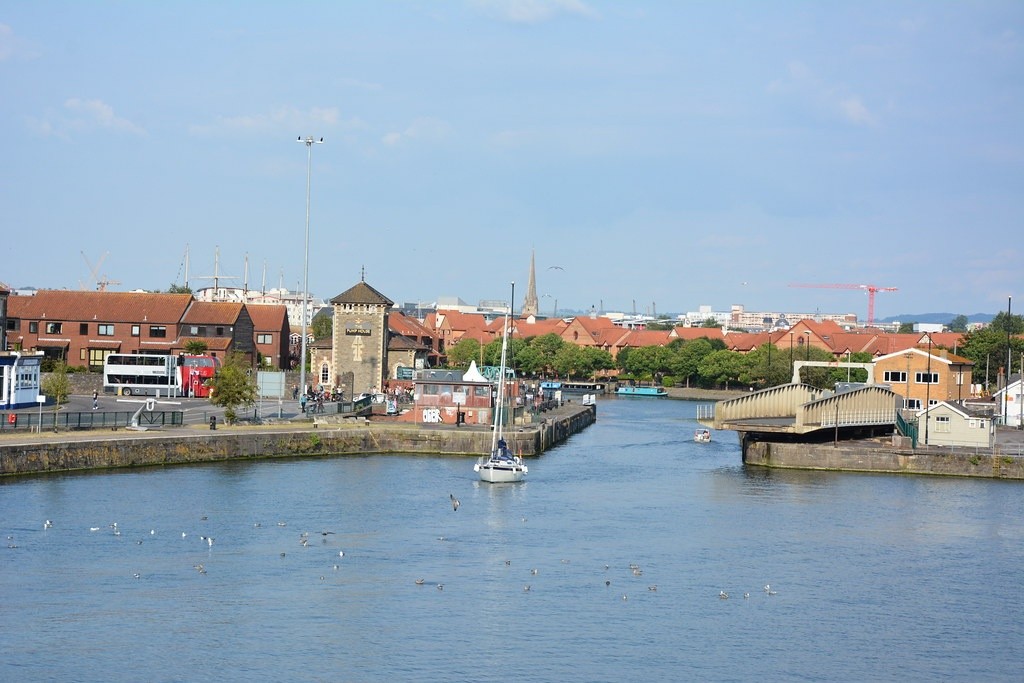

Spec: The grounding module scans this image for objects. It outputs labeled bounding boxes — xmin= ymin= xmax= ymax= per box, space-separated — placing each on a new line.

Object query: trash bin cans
xmin=209 ymin=415 xmax=216 ymax=430
xmin=458 ymin=412 xmax=465 ymax=423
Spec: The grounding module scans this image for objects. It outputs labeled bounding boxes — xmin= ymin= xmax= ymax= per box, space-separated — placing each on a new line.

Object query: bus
xmin=102 ymin=353 xmax=222 ymax=397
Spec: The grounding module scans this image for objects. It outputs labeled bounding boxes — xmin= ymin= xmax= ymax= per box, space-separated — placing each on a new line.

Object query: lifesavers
xmin=11 ymin=415 xmax=15 ymax=423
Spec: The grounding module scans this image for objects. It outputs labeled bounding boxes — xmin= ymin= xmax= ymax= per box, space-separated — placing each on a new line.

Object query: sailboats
xmin=472 ymin=305 xmax=529 ymax=484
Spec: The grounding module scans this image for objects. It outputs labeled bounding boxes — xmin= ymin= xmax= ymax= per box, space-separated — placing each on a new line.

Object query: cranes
xmin=787 ymin=281 xmax=898 ymax=325
xmin=78 ymin=250 xmax=122 ymax=292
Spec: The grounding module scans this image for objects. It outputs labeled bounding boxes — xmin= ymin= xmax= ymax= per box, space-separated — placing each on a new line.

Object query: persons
xmin=372 ymin=385 xmax=414 ymax=402
xmin=315 ymin=393 xmax=324 ymax=413
xmin=291 ymin=384 xmax=342 ymax=401
xmin=93 ymin=388 xmax=99 ymax=409
xmin=301 ymin=394 xmax=306 ymax=412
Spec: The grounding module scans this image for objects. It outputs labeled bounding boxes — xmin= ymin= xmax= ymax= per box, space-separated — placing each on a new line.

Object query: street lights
xmin=295 ymin=135 xmax=324 ymax=407
xmin=767 ymin=329 xmax=812 ymax=383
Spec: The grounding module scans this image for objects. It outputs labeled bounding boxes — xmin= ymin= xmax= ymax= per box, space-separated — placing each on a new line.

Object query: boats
xmin=614 ymin=382 xmax=668 ymax=398
xmin=557 ymin=382 xmax=606 ymax=396
xmin=694 ymin=429 xmax=710 ymax=444
xmin=538 ymin=380 xmax=561 ymax=390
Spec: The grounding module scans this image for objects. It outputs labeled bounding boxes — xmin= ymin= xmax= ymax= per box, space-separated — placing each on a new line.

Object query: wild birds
xmin=200 ymin=535 xmax=217 ymax=546
xmin=529 ymin=567 xmax=538 ymax=574
xmin=319 ymin=575 xmax=326 ymax=580
xmin=132 ymin=573 xmax=141 ymax=578
xmin=339 ymin=550 xmax=349 ymax=559
xmin=414 ymin=576 xmax=425 ymax=585
xmin=137 ymin=539 xmax=144 ymax=545
xmin=278 ymin=520 xmax=287 ymax=527
xmin=603 ymin=561 xmax=660 ymax=602
xmin=331 ymin=563 xmax=340 ymax=569
xmin=560 ymin=558 xmax=571 ymax=564
xmin=181 ymin=531 xmax=188 ymax=537
xmin=201 ymin=515 xmax=209 ymax=520
xmin=718 ymin=583 xmax=779 ymax=601
xmin=192 ymin=563 xmax=208 ymax=574
xmin=299 ymin=530 xmax=310 ymax=548
xmin=440 ymin=536 xmax=446 ymax=541
xmin=108 ymin=521 xmax=121 ymax=536
xmin=523 ymin=584 xmax=531 ymax=591
xmin=89 ymin=526 xmax=100 ymax=531
xmin=436 ymin=583 xmax=444 ymax=589
xmin=547 ymin=265 xmax=564 ymax=271
xmin=315 ymin=531 xmax=336 ymax=535
xmin=279 ymin=551 xmax=286 ymax=557
xmin=150 ymin=529 xmax=155 ymax=535
xmin=4 ymin=534 xmax=18 ymax=549
xmin=449 ymin=493 xmax=461 ymax=512
xmin=253 ymin=522 xmax=263 ymax=527
xmin=43 ymin=519 xmax=54 ymax=529
xmin=504 ymin=559 xmax=512 ymax=568
xmin=521 ymin=515 xmax=528 ymax=522
xmin=541 ymin=294 xmax=552 ymax=299
